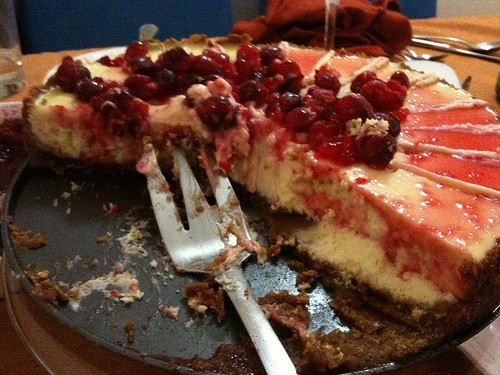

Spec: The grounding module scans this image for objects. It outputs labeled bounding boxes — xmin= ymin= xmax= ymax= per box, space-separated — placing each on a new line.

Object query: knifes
xmin=411 ymin=38 xmax=500 ymax=62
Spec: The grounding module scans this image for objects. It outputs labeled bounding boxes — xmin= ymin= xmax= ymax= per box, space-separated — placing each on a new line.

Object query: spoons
xmin=413 ymin=34 xmax=499 ymax=54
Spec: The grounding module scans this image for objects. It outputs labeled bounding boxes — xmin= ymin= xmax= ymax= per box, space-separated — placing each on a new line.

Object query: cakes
xmin=17 ymin=33 xmax=500 ymax=329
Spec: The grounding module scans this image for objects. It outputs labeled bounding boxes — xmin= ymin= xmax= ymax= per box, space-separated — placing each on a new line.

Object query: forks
xmin=404 ymin=48 xmax=449 ymax=60
xmin=142 ymin=143 xmax=297 ymax=375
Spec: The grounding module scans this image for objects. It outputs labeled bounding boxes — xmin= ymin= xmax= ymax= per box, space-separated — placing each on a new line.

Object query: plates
xmin=1 ymin=148 xmax=499 ymax=375
xmin=42 ymin=45 xmax=129 ymax=86
